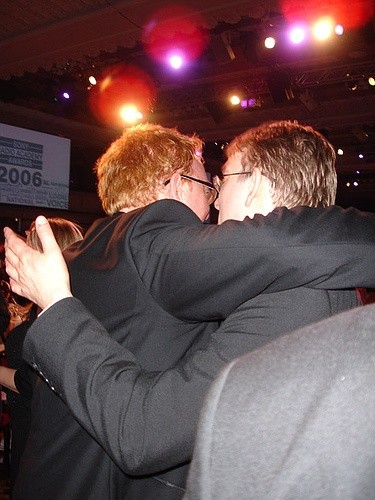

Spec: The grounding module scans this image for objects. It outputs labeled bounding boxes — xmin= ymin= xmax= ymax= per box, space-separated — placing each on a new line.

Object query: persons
xmin=0 ymin=119 xmax=375 ymax=500
xmin=11 ymin=124 xmax=375 ymax=500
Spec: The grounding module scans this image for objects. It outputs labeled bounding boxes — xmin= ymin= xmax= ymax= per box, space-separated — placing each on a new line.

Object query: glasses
xmin=211 ymin=171 xmax=277 ymax=195
xmin=164 ymin=174 xmax=218 ymax=206
